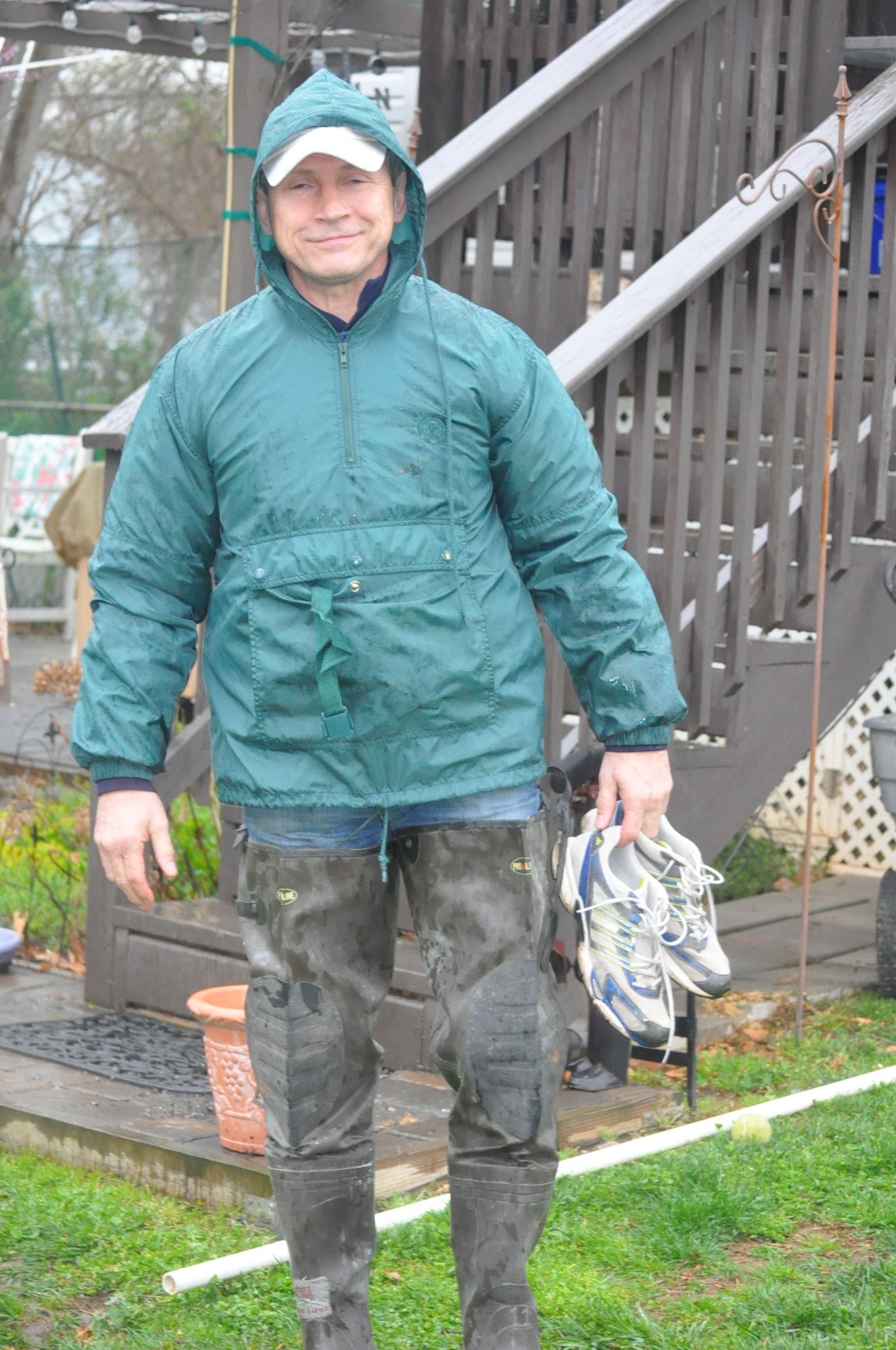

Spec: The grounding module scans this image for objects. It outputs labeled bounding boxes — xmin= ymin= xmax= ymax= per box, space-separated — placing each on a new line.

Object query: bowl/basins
xmin=863 ymin=714 xmax=896 ymax=824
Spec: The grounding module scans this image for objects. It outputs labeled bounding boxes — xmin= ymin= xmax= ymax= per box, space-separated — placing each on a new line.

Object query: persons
xmin=73 ymin=66 xmax=692 ymax=1350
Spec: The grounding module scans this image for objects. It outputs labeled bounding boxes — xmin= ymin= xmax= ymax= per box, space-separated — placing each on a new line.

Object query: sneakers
xmin=580 ymin=795 xmax=733 ymax=1000
xmin=552 ymin=824 xmax=678 ymax=1069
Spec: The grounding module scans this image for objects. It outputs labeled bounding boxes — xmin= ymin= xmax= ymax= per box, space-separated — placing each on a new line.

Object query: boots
xmin=265 ymin=1160 xmax=375 ymax=1350
xmin=444 ymin=1162 xmax=556 ymax=1350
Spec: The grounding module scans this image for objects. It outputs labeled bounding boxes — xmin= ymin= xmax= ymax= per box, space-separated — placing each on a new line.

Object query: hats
xmin=260 ymin=126 xmax=387 ymax=188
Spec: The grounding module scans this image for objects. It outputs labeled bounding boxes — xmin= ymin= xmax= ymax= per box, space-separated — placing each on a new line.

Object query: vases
xmin=186 ymin=985 xmax=266 ymax=1157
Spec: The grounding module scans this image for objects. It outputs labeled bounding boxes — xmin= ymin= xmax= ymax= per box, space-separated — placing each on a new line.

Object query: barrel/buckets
xmin=870 ymin=162 xmax=889 ymax=275
xmin=186 ymin=985 xmax=266 ymax=1155
